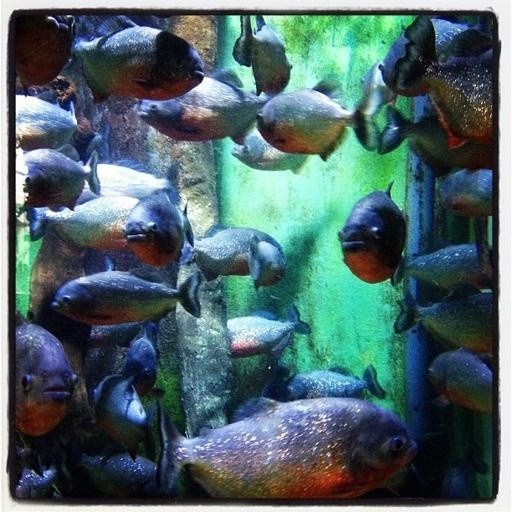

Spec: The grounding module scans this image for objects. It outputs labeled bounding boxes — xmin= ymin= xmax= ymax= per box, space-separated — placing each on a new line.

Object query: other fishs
xmin=12 ymin=12 xmax=494 ymax=502
